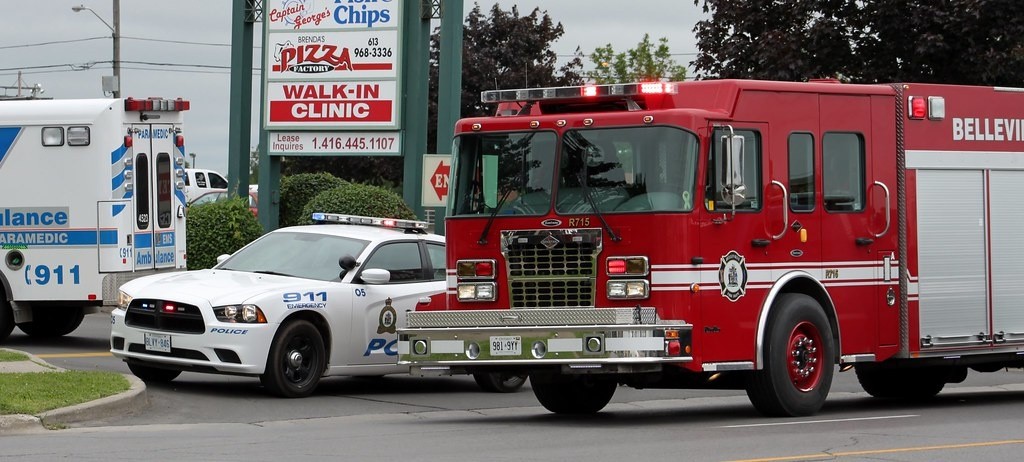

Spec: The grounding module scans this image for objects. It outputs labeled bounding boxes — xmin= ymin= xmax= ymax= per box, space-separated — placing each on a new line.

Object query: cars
xmin=184 ymin=169 xmax=258 ymax=218
xmin=110 ymin=212 xmax=446 ymax=398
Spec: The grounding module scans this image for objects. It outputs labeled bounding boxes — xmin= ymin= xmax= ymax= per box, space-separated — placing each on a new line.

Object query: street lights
xmin=189 ymin=153 xmax=196 ymax=168
xmin=71 ymin=4 xmax=122 ymax=99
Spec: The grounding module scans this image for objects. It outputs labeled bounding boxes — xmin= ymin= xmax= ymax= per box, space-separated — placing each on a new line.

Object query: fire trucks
xmin=397 ymin=78 xmax=1024 ymax=417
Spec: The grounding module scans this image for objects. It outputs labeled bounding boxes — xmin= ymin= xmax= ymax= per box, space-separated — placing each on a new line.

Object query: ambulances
xmin=0 ymin=97 xmax=190 ymax=345
